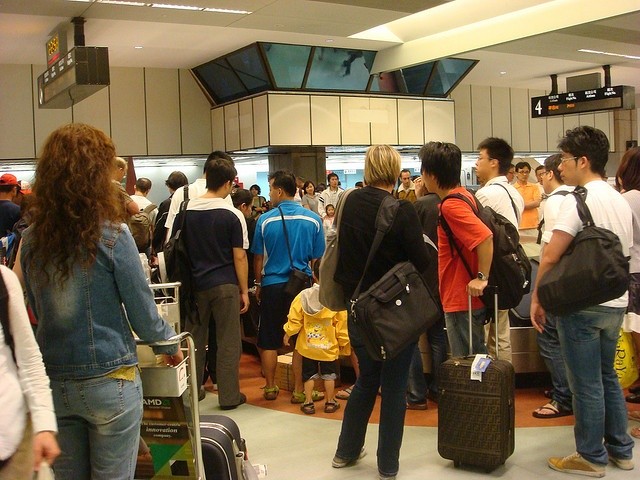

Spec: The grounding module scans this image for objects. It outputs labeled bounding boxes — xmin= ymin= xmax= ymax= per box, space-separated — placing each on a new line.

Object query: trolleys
xmin=146 ymin=281 xmax=181 ymax=336
xmin=131 ymin=332 xmax=205 ymax=479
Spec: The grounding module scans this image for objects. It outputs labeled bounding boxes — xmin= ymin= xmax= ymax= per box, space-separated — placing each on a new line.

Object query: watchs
xmin=253 ymin=279 xmax=261 ymax=286
xmin=477 ymin=271 xmax=490 ymax=281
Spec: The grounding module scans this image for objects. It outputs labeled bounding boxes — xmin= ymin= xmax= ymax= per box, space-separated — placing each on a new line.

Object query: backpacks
xmin=434 ymin=188 xmax=531 ymax=310
xmin=164 ymin=199 xmax=203 ymax=326
xmin=0 ymin=229 xmax=22 ymax=271
xmin=127 ymin=204 xmax=157 ymax=251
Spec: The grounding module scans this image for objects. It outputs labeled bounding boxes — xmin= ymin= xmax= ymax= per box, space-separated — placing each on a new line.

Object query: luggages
xmin=197 ymin=415 xmax=248 ymax=480
xmin=437 ymin=286 xmax=516 ymax=473
xmin=508 ymin=257 xmax=540 ymax=327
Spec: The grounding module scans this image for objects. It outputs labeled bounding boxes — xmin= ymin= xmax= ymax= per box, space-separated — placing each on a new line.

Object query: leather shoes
xmin=626 ymin=393 xmax=640 ymax=404
xmin=628 ymin=385 xmax=639 ymax=393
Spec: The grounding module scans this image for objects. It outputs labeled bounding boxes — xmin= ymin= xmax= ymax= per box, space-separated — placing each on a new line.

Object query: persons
xmin=334 ymin=348 xmax=381 ymax=401
xmin=164 ymin=151 xmax=236 ymax=391
xmin=318 ymin=172 xmax=344 ymax=219
xmin=418 ymin=141 xmax=494 ymax=357
xmin=342 ymin=50 xmax=363 ymax=76
xmin=130 ymin=177 xmax=159 ymax=253
xmin=510 ymin=161 xmax=542 ymax=257
xmin=392 ymin=169 xmax=417 ymax=204
xmin=249 ymin=184 xmax=269 ymax=220
xmin=332 ymin=144 xmax=432 ymax=480
xmin=11 ymin=123 xmax=182 ymax=480
xmin=293 ymin=188 xmax=303 ymax=206
xmin=395 ymin=167 xmax=448 ymax=411
xmin=476 ymin=177 xmax=485 ymax=190
xmin=535 ymin=165 xmax=546 ymax=220
xmin=318 ymin=47 xmax=337 ymax=60
xmin=251 ymin=170 xmax=326 ymax=404
xmin=618 ymin=146 xmax=640 ymax=404
xmin=285 ymin=258 xmax=352 ymax=414
xmin=0 ymin=264 xmax=60 ymax=480
xmin=601 ymin=169 xmax=608 ymax=182
xmin=109 ymin=157 xmax=139 ymax=223
xmin=505 ymin=163 xmax=515 ymax=184
xmin=170 ymin=157 xmax=249 ymax=411
xmin=614 ymin=170 xmax=626 ymax=194
xmin=301 ymin=180 xmax=320 ymax=214
xmin=547 ymin=125 xmax=635 ymax=478
xmin=12 ymin=180 xmax=33 ymax=206
xmin=232 ymin=190 xmax=258 ymax=289
xmin=474 ymin=136 xmax=525 ymax=364
xmin=322 ymin=204 xmax=335 ymax=235
xmin=627 ymin=410 xmax=640 ymax=440
xmin=532 ymin=153 xmax=576 ymax=418
xmin=355 ymin=181 xmax=363 ymax=189
xmin=0 ymin=174 xmax=21 ymax=265
xmin=230 ymin=181 xmax=240 ymax=198
xmin=296 ymin=175 xmax=305 ymax=189
xmin=152 ymin=171 xmax=188 ymax=255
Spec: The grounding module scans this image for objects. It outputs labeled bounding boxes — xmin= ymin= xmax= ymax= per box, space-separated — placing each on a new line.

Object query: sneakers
xmin=198 ymin=389 xmax=206 ymax=402
xmin=332 ymin=447 xmax=367 ymax=469
xmin=403 ymin=397 xmax=427 ymax=409
xmin=547 ymin=452 xmax=606 ymax=478
xmin=608 ymin=455 xmax=635 ymax=470
xmin=221 ymin=391 xmax=246 ymax=410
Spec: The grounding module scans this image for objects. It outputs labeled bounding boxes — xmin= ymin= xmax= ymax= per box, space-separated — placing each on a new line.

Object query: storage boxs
xmin=274 ymin=350 xmax=343 ymax=392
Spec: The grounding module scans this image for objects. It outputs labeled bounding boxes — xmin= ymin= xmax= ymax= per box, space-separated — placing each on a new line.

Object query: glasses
xmin=560 ymin=158 xmax=577 ymax=166
xmin=476 ymin=155 xmax=494 ymax=161
xmin=518 ymin=171 xmax=530 ymax=174
xmin=538 ymin=171 xmax=549 ymax=179
xmin=508 ymin=171 xmax=514 ymax=175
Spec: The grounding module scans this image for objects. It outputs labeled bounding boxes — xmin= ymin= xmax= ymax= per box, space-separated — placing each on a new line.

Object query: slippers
xmin=334 ymin=387 xmax=352 ymax=401
xmin=532 ymin=400 xmax=573 ymax=418
xmin=545 ymin=388 xmax=556 ymax=398
xmin=544 ymin=391 xmax=555 ymax=399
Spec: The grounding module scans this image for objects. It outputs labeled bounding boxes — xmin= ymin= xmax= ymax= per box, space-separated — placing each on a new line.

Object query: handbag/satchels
xmin=290 ymin=266 xmax=312 ymax=292
xmin=613 ymin=328 xmax=639 ymax=390
xmin=319 ymin=229 xmax=347 ymax=312
xmin=349 ymin=260 xmax=442 ymax=362
xmin=536 ymin=227 xmax=632 ymax=319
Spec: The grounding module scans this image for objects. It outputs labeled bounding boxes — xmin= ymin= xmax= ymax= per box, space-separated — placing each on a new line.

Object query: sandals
xmin=630 ymin=426 xmax=640 ymax=439
xmin=263 ymin=384 xmax=280 ymax=400
xmin=324 ymin=400 xmax=340 ymax=413
xmin=627 ymin=410 xmax=640 ymax=422
xmin=290 ymin=390 xmax=324 ymax=404
xmin=301 ymin=402 xmax=315 ymax=414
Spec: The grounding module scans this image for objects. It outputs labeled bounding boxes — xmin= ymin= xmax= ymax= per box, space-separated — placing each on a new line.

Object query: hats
xmin=17 ymin=180 xmax=33 ymax=195
xmin=0 ymin=174 xmax=21 ymax=188
